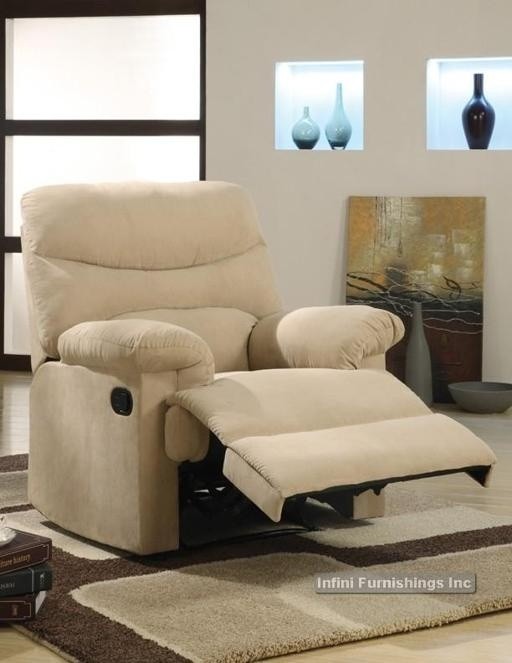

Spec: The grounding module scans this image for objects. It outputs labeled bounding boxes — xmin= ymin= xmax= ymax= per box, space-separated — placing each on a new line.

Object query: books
xmin=0 ymin=527 xmax=54 ymax=623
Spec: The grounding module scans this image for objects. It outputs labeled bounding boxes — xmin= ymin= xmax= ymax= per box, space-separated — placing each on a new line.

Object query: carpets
xmin=0 ymin=451 xmax=512 ymax=663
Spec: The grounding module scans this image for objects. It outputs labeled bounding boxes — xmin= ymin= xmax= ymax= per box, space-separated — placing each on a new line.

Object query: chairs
xmin=20 ymin=179 xmax=497 ymax=559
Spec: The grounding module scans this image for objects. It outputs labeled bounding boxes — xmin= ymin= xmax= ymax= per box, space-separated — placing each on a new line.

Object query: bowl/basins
xmin=446 ymin=381 xmax=512 ymax=414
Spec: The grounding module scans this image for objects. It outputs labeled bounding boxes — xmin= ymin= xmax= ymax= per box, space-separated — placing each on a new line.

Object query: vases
xmin=405 ymin=301 xmax=433 ymax=408
xmin=292 ymin=106 xmax=320 ymax=150
xmin=324 ymin=82 xmax=352 ymax=150
xmin=462 ymin=74 xmax=496 ymax=150
